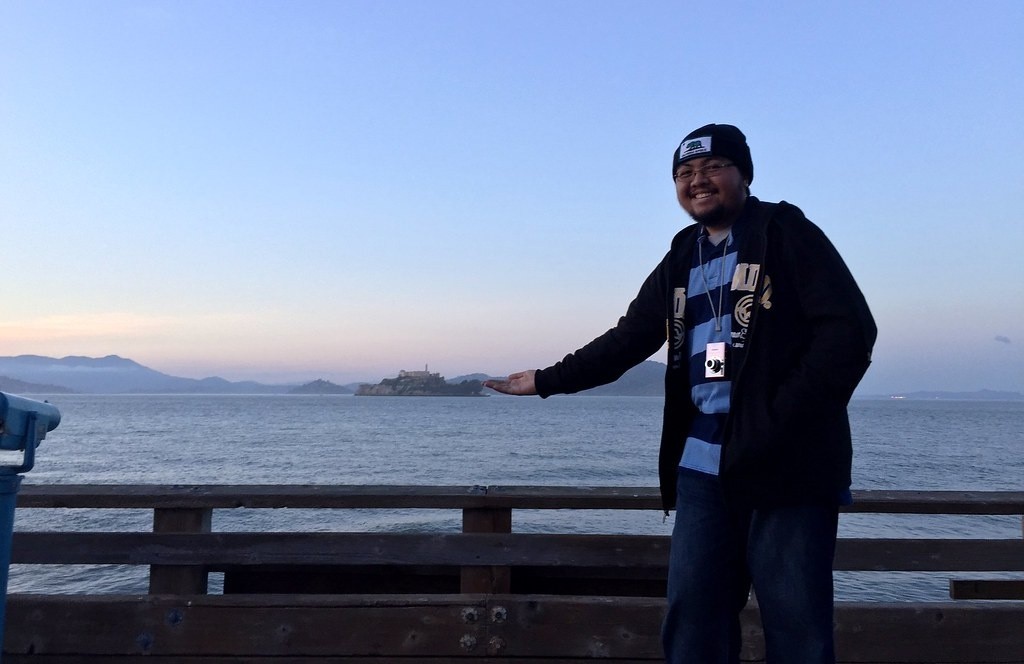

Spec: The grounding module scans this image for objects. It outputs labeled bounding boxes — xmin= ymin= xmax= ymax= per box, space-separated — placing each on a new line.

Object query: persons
xmin=481 ymin=122 xmax=878 ymax=664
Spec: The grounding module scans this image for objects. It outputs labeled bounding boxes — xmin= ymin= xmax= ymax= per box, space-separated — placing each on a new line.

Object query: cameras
xmin=705 ymin=342 xmax=727 ymax=378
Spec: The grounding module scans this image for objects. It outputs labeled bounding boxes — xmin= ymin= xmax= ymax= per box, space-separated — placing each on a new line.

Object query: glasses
xmin=673 ymin=163 xmax=737 ymax=181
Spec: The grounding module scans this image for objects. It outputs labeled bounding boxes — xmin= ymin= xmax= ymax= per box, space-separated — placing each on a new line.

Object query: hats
xmin=672 ymin=124 xmax=754 ymax=186
